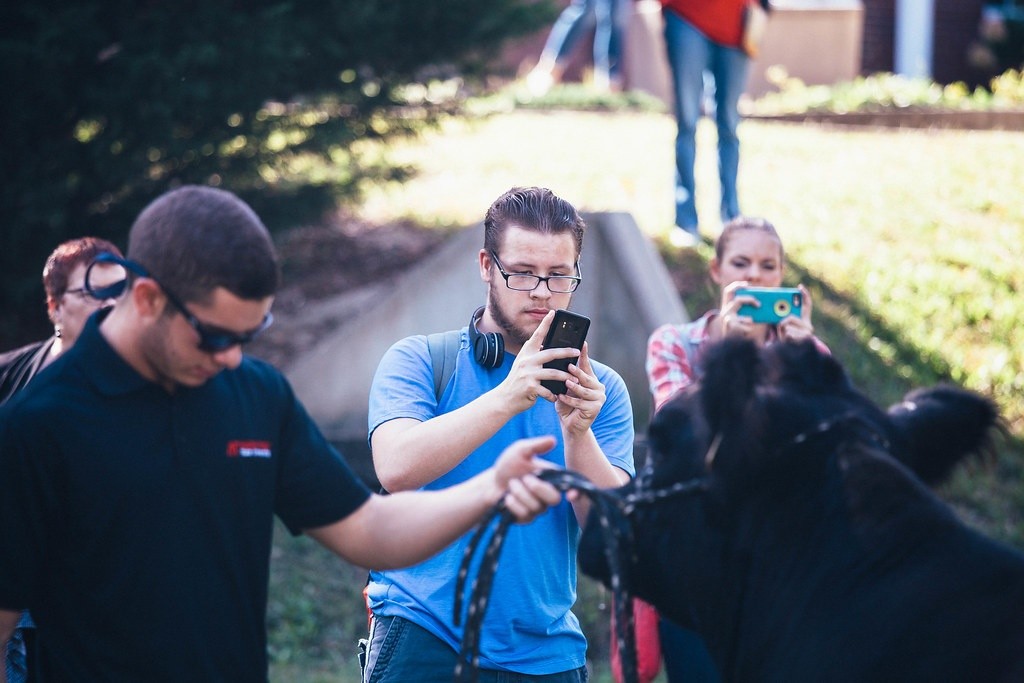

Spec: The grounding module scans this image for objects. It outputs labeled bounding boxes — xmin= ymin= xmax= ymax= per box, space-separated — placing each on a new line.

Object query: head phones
xmin=467 ymin=305 xmax=505 ymax=370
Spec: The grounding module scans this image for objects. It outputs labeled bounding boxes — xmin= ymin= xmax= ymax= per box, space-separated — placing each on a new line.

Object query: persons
xmin=0 ymin=183 xmax=584 ymax=683
xmin=659 ymin=1 xmax=770 ymax=244
xmin=0 ymin=237 xmax=127 ymax=683
xmin=605 ymin=210 xmax=833 ymax=682
xmin=529 ymin=0 xmax=626 ymax=93
xmin=357 ymin=186 xmax=637 ymax=683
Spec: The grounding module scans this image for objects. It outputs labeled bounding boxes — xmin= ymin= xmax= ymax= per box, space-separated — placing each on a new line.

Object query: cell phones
xmin=736 ymin=287 xmax=803 ymax=325
xmin=540 ymin=309 xmax=591 ymax=396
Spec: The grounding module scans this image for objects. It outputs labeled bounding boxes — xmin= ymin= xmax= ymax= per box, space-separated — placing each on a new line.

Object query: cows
xmin=578 ymin=330 xmax=1022 ymax=682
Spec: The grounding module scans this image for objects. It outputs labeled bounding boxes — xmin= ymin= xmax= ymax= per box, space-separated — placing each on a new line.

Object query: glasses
xmin=491 ymin=251 xmax=581 ymax=293
xmin=162 ymin=292 xmax=275 ymax=352
xmin=67 ymin=286 xmax=112 ymax=305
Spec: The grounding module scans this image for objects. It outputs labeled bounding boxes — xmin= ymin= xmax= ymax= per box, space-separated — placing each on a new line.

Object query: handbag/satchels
xmin=741 ymin=3 xmax=768 ymax=59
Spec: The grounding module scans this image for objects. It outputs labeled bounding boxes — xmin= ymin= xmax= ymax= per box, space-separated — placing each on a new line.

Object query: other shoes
xmin=669 ymin=225 xmax=699 ymax=248
